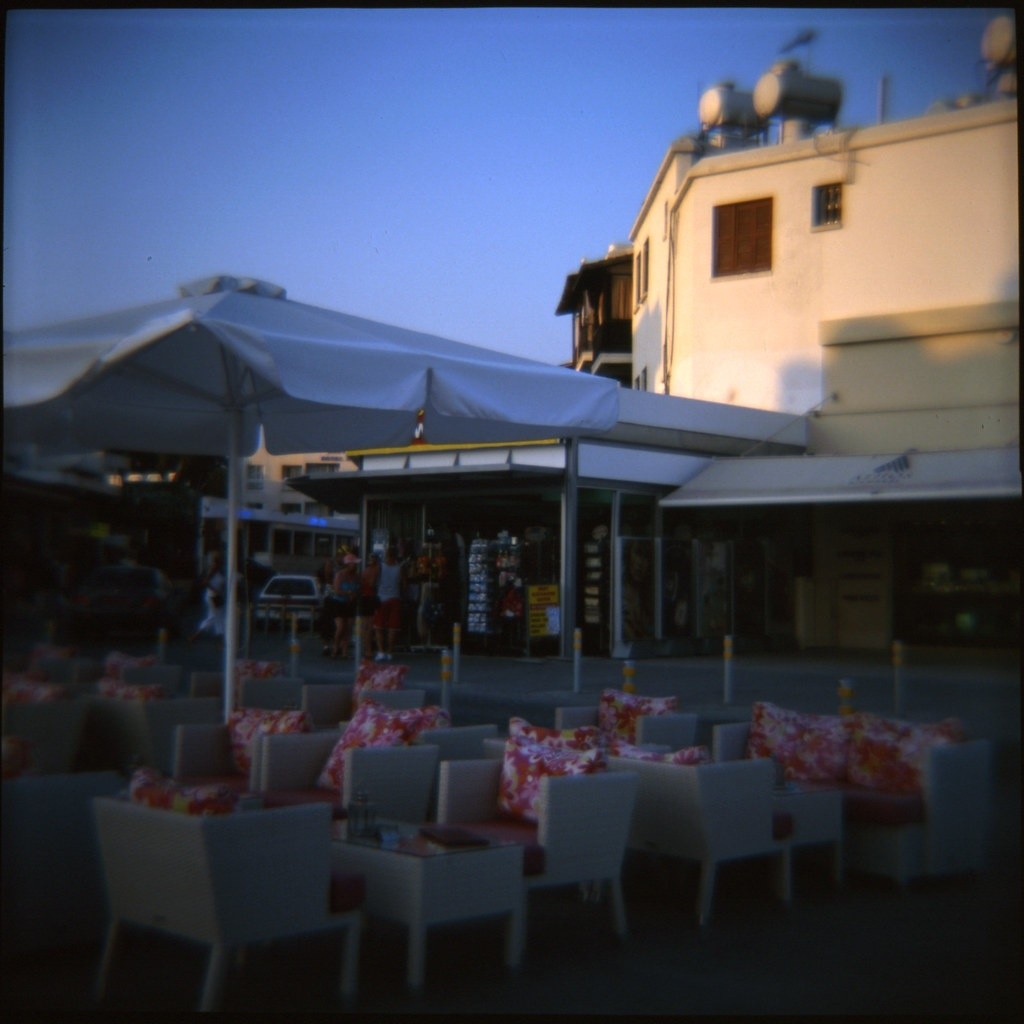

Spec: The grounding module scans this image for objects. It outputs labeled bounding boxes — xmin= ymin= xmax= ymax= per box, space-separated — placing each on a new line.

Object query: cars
xmin=63 ymin=561 xmax=182 ymax=642
xmin=256 ymin=571 xmax=332 ymax=630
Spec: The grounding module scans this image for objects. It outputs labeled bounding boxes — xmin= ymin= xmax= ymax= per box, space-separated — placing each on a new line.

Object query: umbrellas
xmin=0 ymin=273 xmax=621 ymax=727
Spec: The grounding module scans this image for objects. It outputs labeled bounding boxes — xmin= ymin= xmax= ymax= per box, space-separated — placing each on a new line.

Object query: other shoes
xmin=375 ymin=651 xmax=393 ymax=662
xmin=331 ymin=651 xmax=354 ymax=660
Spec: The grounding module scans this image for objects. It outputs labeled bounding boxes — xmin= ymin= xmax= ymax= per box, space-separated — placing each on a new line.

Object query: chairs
xmin=1 ymin=653 xmax=999 ymax=1012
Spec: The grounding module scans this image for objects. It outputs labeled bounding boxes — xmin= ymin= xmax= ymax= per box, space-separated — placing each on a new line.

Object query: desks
xmin=325 ymin=823 xmax=528 ymax=995
xmin=762 ymin=779 xmax=851 ymax=882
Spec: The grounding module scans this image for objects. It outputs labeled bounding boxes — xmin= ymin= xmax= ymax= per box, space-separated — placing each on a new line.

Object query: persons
xmin=324 ymin=552 xmax=362 ymax=659
xmin=192 ymin=561 xmax=224 ymax=639
xmin=372 ymin=544 xmax=407 ymax=659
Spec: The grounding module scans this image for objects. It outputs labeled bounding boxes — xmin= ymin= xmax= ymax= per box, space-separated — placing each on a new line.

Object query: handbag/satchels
xmin=416 ymin=525 xmax=445 ymax=580
xmin=211 ymin=594 xmax=224 ymax=607
xmin=495 ymin=538 xmax=525 ymax=620
xmin=424 ymin=590 xmax=451 ymax=629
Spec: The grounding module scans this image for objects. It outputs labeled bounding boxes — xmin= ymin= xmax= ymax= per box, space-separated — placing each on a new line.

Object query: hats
xmin=344 ymin=553 xmax=362 ymax=565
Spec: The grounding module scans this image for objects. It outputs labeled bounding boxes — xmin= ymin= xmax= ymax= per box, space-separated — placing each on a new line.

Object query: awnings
xmin=658 ymin=446 xmax=1023 ymax=507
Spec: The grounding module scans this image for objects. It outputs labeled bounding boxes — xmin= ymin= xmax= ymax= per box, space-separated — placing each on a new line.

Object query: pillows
xmin=1 ymin=643 xmax=958 ymax=819
